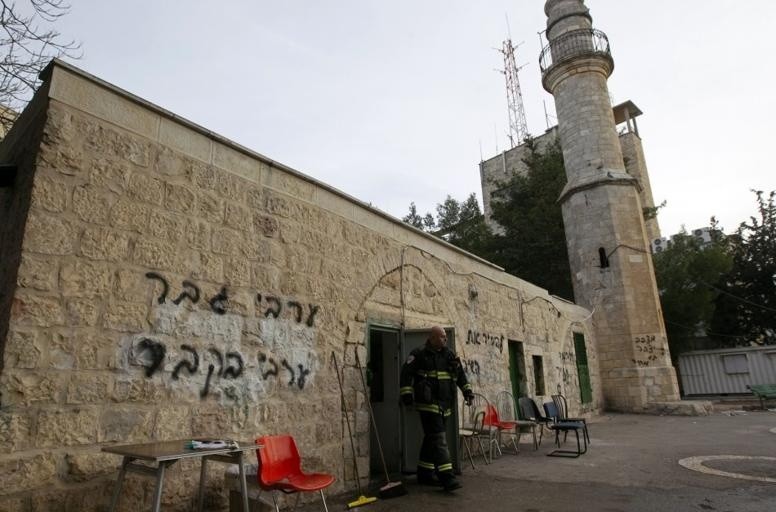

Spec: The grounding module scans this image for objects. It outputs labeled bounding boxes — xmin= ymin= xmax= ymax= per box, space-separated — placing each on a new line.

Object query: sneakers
xmin=417 ymin=475 xmax=441 ymax=486
xmin=443 ymin=478 xmax=464 ymax=493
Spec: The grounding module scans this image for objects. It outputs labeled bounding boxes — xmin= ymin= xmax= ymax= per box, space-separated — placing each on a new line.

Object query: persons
xmin=400 ymin=325 xmax=474 ymax=489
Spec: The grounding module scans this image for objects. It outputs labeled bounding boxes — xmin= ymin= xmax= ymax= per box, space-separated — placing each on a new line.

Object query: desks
xmin=100 ymin=437 xmax=267 ymax=511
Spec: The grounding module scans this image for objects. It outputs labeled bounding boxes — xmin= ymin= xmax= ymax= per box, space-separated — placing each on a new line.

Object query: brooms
xmin=354 ymin=347 xmax=407 ymax=500
xmin=333 ymin=350 xmax=378 ymax=508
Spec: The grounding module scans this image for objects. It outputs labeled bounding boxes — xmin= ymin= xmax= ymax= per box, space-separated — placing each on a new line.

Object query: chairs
xmin=459 ymin=390 xmax=591 ymax=471
xmin=250 ymin=434 xmax=337 ymax=512
